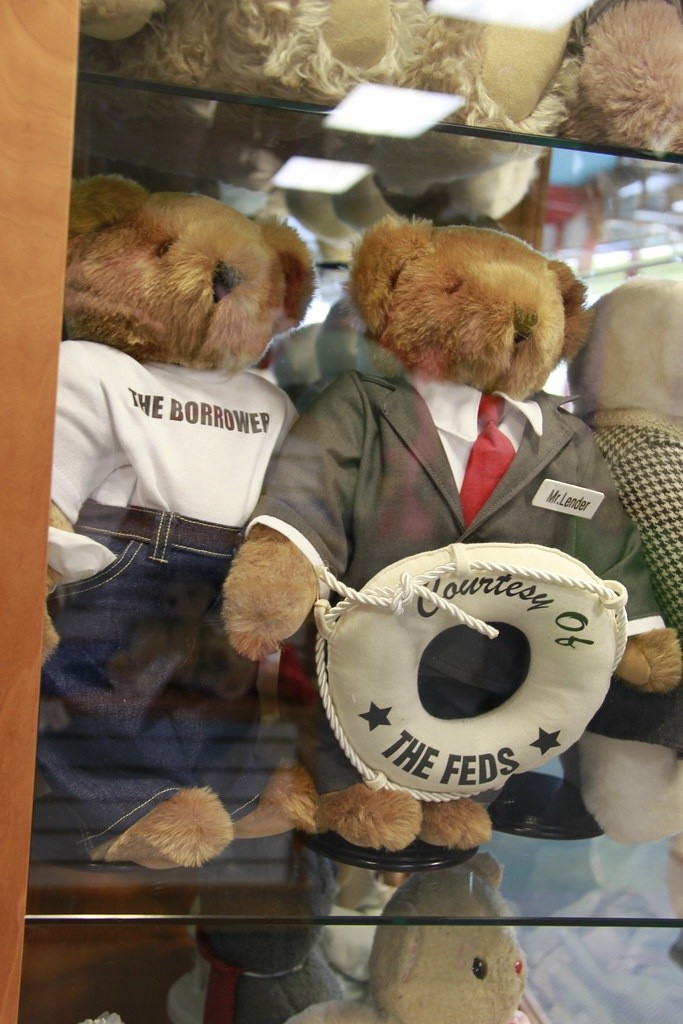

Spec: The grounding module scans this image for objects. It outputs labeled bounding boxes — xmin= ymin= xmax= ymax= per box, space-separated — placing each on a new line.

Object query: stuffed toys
xmin=220 ymin=213 xmax=683 ymax=852
xmin=28 ymin=2 xmax=681 ymax=1023
xmin=36 ymin=173 xmax=330 ymax=867
